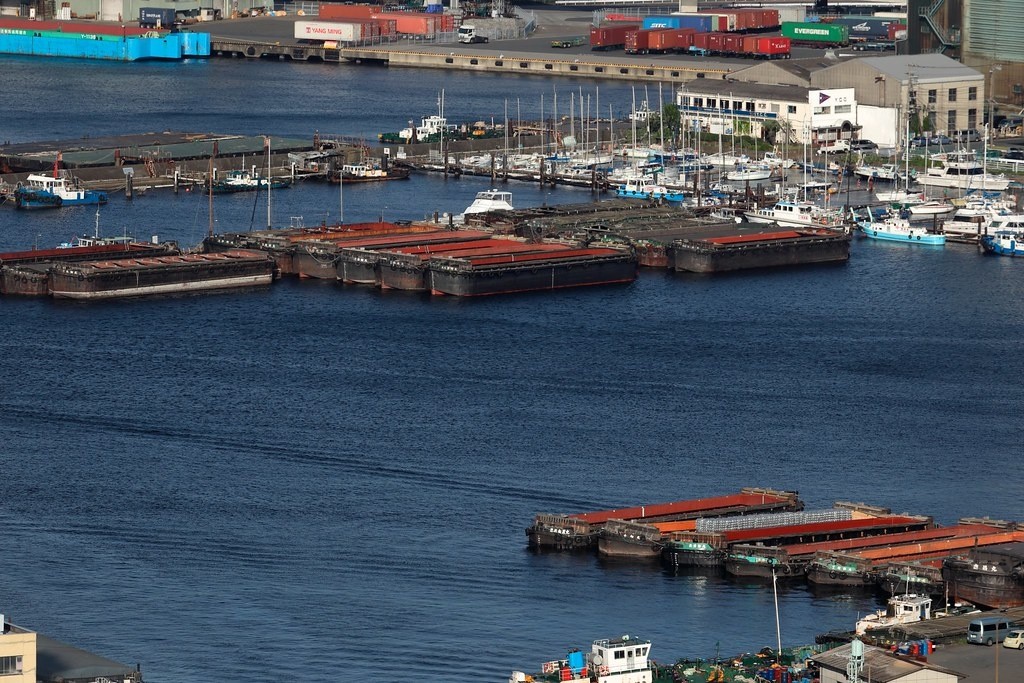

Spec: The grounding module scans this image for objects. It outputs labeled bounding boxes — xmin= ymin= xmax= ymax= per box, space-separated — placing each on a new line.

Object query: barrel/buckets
xmin=891 ymin=639 xmax=932 ymax=655
xmin=762 ymin=667 xmax=788 ymax=683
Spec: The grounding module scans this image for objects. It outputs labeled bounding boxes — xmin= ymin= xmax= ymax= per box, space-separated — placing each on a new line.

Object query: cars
xmin=912 ymin=135 xmax=952 ymax=146
xmin=1003 ymin=630 xmax=1024 ymax=650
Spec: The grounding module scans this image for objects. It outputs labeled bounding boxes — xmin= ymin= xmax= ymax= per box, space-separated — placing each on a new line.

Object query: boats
xmin=525 ymin=486 xmax=1024 ymax=609
xmin=464 ymin=188 xmax=515 ymax=216
xmin=853 ymin=122 xmax=1024 ymax=236
xmin=614 ymin=175 xmax=685 ymax=202
xmin=329 ymin=155 xmax=413 ymax=185
xmin=55 ymin=204 xmax=137 ymax=250
xmin=397 ymin=89 xmax=448 ymax=141
xmin=976 ymin=227 xmax=1024 ymax=261
xmin=0 ymin=240 xmax=274 ymax=309
xmin=727 ymin=138 xmax=772 ymax=182
xmin=14 ymin=156 xmax=107 ymax=211
xmin=202 ymin=154 xmax=292 ymax=195
xmin=193 ymin=136 xmax=851 ymax=300
xmin=856 ymin=566 xmax=982 ymax=638
xmin=857 ymin=211 xmax=946 ymax=246
xmin=677 ymin=112 xmax=851 ymax=235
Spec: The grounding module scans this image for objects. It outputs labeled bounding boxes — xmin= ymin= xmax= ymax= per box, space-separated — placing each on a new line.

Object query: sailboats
xmin=458 ymin=80 xmax=699 ymax=185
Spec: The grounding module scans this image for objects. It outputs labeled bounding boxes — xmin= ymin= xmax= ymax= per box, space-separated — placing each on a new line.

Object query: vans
xmin=953 ymin=129 xmax=981 ymax=143
xmin=851 ymin=139 xmax=878 ymax=150
xmin=967 ymin=618 xmax=1016 ymax=646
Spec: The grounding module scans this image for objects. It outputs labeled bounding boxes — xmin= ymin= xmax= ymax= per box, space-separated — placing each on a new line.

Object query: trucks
xmin=458 ymin=25 xmax=489 ymax=44
xmin=820 ymin=140 xmax=850 ymax=155
